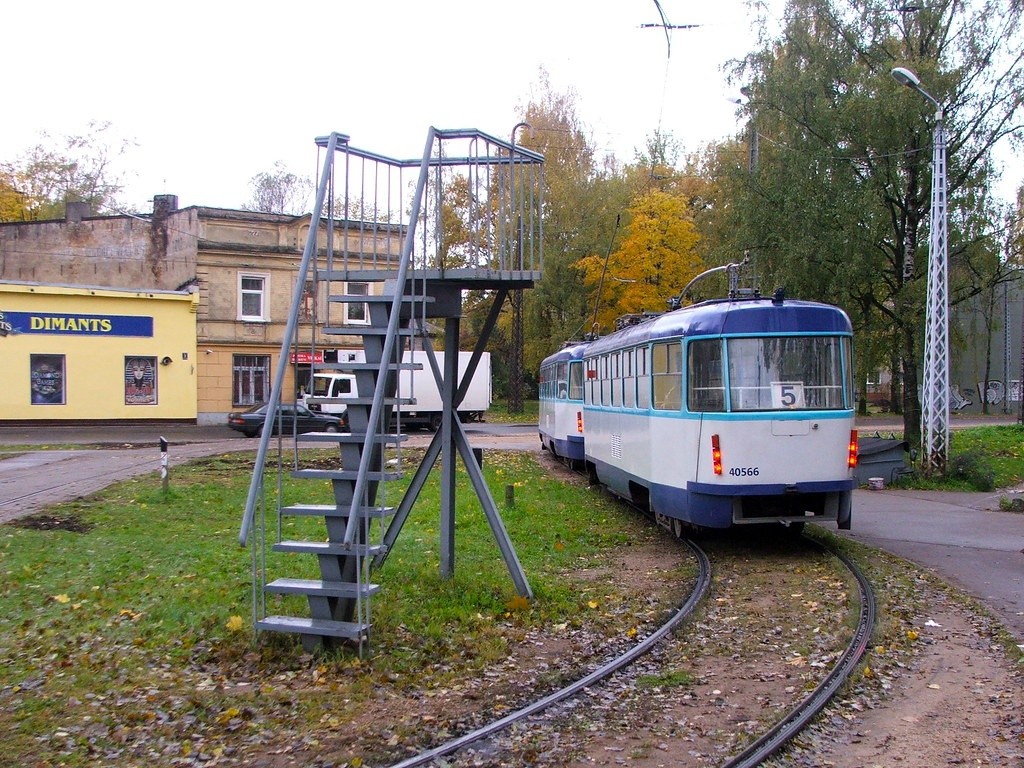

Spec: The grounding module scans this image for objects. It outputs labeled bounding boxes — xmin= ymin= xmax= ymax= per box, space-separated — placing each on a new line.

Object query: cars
xmin=227 ymin=401 xmax=344 ymax=438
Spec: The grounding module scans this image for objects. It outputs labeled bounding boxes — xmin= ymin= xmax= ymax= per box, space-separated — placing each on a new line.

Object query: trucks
xmin=300 ymin=347 xmax=493 ymax=432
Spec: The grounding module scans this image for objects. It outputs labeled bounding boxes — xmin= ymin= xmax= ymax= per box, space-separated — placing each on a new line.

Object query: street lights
xmin=889 ymin=66 xmax=952 ymax=478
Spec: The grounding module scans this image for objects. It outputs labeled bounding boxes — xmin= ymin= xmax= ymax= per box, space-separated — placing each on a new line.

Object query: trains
xmin=534 ymin=289 xmax=861 ymax=542
